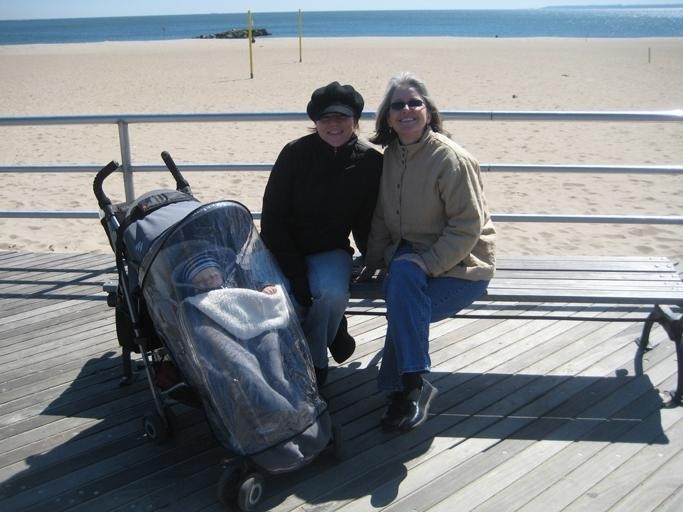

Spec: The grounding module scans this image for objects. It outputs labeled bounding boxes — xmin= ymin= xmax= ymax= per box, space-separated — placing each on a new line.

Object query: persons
xmin=183 ymin=254 xmax=277 ymax=296
xmin=359 ymin=73 xmax=497 ymax=435
xmin=258 ymin=81 xmax=384 ymax=391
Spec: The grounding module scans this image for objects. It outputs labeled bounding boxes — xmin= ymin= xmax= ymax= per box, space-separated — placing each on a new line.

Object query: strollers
xmin=88 ymin=150 xmax=340 ymax=512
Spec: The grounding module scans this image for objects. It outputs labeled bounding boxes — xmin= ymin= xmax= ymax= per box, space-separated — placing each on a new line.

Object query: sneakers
xmin=329 ymin=315 xmax=355 ymax=363
xmin=381 ymin=377 xmax=438 ymax=431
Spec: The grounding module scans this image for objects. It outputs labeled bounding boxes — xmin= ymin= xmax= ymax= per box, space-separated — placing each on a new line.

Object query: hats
xmin=182 ymin=252 xmax=222 ymax=284
xmin=307 ymin=81 xmax=364 ymax=121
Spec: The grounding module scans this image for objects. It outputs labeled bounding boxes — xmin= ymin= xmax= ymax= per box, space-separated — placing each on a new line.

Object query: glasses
xmin=391 ymin=100 xmax=422 ymax=110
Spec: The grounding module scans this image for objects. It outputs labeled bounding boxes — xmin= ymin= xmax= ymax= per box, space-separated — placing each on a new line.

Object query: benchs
xmin=100 ymin=252 xmax=683 ymax=411
